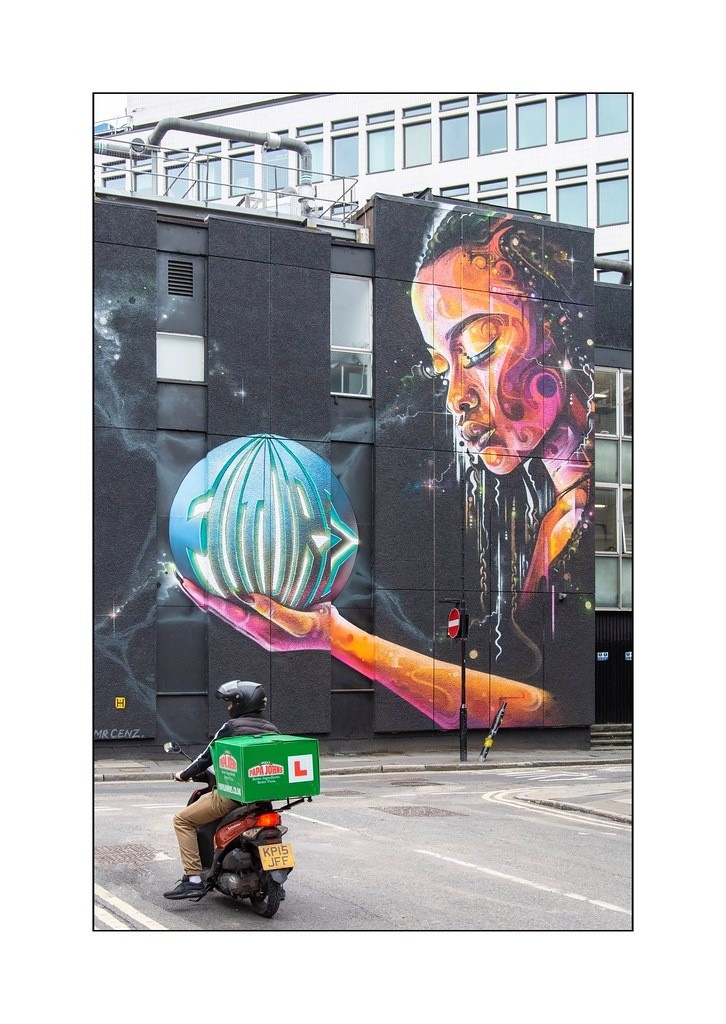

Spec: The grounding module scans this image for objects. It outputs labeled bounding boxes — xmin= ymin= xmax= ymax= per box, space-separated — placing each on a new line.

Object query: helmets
xmin=215 ymin=681 xmax=268 ymax=719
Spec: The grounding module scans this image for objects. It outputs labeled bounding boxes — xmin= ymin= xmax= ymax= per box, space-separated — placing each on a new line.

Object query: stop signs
xmin=448 ymin=608 xmax=461 ymax=639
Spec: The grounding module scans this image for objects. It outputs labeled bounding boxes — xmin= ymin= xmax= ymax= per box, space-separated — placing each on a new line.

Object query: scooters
xmin=163 ymin=740 xmax=313 ymax=918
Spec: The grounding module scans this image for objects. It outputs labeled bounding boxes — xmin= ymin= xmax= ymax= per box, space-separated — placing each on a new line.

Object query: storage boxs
xmin=209 ymin=732 xmax=320 ymax=804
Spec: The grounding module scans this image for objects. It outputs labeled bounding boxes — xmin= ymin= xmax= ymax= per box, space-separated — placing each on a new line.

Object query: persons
xmin=163 ymin=680 xmax=282 ymax=901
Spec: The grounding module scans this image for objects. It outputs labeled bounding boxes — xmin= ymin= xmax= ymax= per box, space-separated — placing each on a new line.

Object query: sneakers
xmin=163 ymin=874 xmax=207 ymax=900
